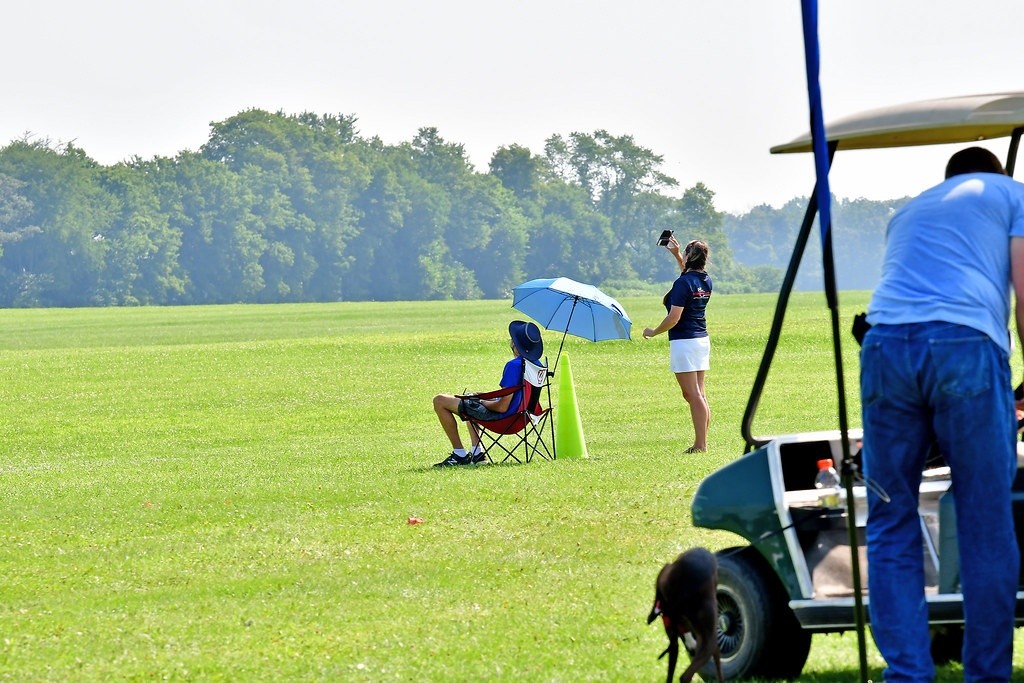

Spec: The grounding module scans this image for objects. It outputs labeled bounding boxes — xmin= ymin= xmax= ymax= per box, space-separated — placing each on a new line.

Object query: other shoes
xmin=683 ymin=446 xmax=697 ymax=454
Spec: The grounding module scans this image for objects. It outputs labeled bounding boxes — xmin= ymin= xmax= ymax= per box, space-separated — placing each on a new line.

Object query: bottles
xmin=814 ymin=459 xmax=842 ymax=518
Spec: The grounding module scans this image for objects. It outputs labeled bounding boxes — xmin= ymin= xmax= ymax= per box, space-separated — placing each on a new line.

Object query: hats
xmin=509 ymin=321 xmax=544 ymax=361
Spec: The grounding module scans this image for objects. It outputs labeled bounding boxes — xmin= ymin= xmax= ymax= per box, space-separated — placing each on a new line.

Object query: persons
xmin=643 ymin=234 xmax=712 ymax=454
xmin=851 ymin=147 xmax=1023 ymax=683
xmin=434 ymin=320 xmax=546 ymax=468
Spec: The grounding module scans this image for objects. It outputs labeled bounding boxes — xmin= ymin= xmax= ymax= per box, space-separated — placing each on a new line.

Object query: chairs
xmin=455 ymin=356 xmax=556 ymax=465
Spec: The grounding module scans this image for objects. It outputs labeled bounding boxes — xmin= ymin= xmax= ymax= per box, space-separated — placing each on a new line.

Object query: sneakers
xmin=434 ymin=451 xmax=473 ymax=469
xmin=472 ymin=452 xmax=486 ymax=465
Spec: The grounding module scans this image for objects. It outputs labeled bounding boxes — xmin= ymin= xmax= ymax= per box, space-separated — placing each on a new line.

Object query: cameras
xmin=656 ymin=230 xmax=675 ymax=247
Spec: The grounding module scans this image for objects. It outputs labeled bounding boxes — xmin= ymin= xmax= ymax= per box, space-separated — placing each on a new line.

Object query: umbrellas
xmin=511 ymin=277 xmax=633 ymax=373
